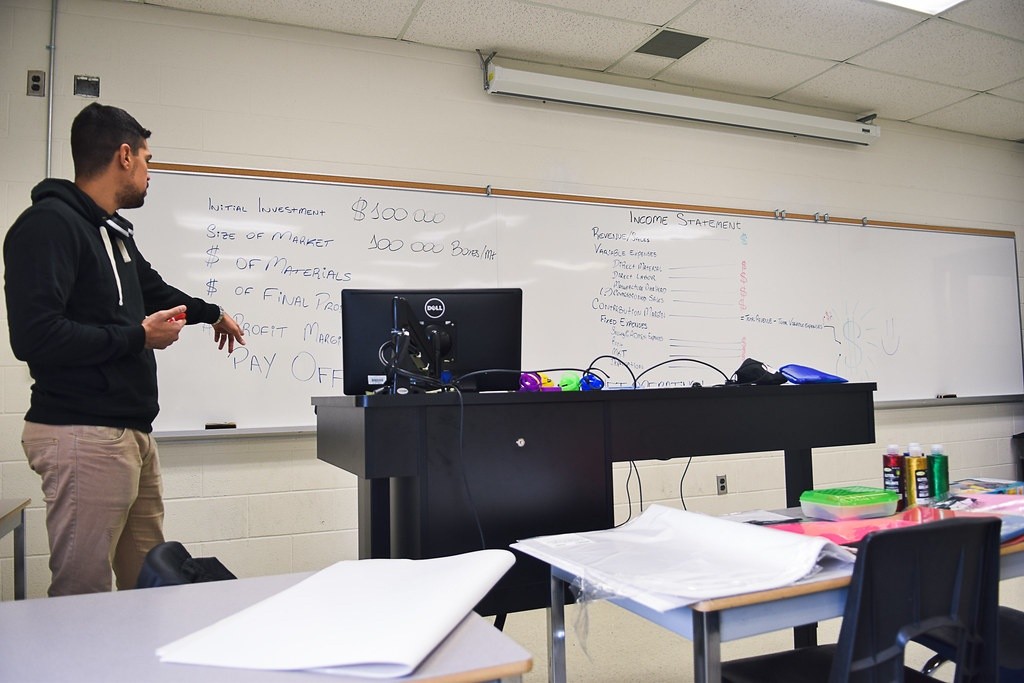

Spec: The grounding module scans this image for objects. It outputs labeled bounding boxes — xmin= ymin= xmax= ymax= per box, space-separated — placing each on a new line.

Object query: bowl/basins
xmin=799 ymin=485 xmax=899 ymax=521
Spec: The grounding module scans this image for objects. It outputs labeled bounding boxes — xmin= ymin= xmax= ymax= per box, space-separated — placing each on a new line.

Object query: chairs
xmin=721 ymin=516 xmax=1001 ymax=683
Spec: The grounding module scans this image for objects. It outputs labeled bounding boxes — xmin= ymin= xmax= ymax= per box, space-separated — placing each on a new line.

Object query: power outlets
xmin=717 ymin=474 xmax=728 ymax=494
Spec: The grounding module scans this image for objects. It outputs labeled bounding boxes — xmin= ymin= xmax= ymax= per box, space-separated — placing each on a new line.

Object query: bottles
xmin=883 ymin=441 xmax=950 ymax=513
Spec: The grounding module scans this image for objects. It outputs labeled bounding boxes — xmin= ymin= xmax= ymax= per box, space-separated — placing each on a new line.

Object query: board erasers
xmin=205 ymin=422 xmax=235 ymax=430
xmin=936 ymin=394 xmax=956 ymax=398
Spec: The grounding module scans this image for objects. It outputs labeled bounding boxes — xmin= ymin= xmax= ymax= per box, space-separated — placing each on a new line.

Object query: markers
xmin=166 ymin=312 xmax=186 ymax=323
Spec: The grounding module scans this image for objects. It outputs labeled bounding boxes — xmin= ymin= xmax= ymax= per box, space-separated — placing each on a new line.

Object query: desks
xmin=0 ymin=569 xmax=534 ymax=683
xmin=551 ymin=476 xmax=1024 ymax=683
xmin=310 ymin=381 xmax=880 ymax=558
xmin=0 ymin=497 xmax=32 ymax=600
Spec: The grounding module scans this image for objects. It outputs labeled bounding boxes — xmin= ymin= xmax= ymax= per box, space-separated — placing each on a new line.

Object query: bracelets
xmin=211 ymin=305 xmax=223 ymax=325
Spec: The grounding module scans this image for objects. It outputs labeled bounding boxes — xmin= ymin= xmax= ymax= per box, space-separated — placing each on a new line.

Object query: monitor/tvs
xmin=342 ymin=288 xmax=522 ymax=395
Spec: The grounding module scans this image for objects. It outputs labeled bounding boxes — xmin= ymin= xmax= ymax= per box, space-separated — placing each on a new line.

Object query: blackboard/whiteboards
xmin=117 ymin=160 xmax=1023 ymax=440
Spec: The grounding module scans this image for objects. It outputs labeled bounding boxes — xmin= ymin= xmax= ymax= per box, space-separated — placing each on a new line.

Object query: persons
xmin=3 ymin=105 xmax=246 ymax=597
